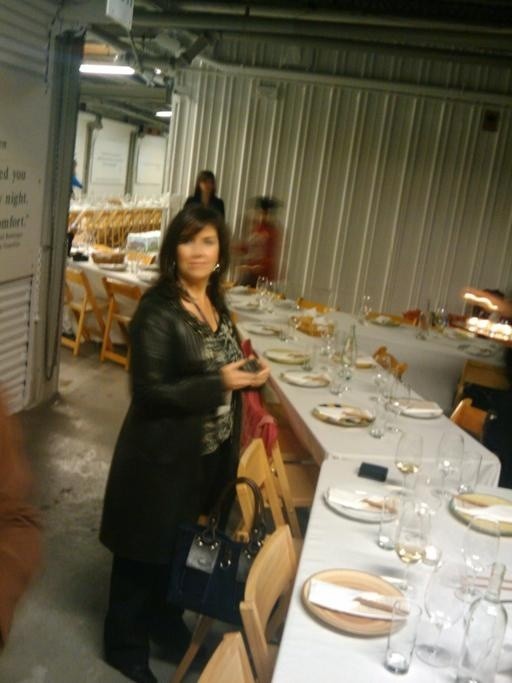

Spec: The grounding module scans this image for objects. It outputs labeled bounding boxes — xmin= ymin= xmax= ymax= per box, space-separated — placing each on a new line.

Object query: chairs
xmin=60 ymin=194 xmax=163 ymax=371
xmin=173 ymin=391 xmax=512 ymax=682
xmin=451 ymin=360 xmax=511 ymax=408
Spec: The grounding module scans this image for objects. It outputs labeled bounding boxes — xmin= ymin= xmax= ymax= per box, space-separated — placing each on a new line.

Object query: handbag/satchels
xmin=179 ymin=524 xmax=280 ymax=627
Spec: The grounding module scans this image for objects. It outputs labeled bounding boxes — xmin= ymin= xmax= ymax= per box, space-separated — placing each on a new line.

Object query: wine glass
xmin=224 ymin=273 xmax=512 ymax=683
xmin=69 ymin=189 xmax=166 ymax=208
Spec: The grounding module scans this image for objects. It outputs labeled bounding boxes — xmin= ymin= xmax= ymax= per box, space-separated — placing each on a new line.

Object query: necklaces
xmin=191 ymin=298 xmax=219 ymax=330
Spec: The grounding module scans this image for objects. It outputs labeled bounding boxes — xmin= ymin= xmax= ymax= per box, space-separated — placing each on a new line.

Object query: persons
xmin=183 ymin=169 xmax=225 ymax=219
xmin=230 ymin=194 xmax=280 ymax=289
xmin=70 ymin=160 xmax=83 ymax=200
xmin=97 ymin=202 xmax=270 ymax=681
xmin=1 ymin=390 xmax=46 ymax=649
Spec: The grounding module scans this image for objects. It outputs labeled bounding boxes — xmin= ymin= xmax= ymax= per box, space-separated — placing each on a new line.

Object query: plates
xmin=65 ymin=226 xmax=161 ymax=285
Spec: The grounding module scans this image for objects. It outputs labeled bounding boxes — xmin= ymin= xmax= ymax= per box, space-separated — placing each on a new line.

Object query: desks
xmin=235 ymin=318 xmax=500 ymax=485
xmin=226 ymin=284 xmax=512 ymax=413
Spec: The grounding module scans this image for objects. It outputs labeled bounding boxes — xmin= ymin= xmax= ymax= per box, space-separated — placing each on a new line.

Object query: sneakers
xmin=154 ymin=628 xmax=210 ymax=659
xmin=105 ymin=650 xmax=159 ymax=683
